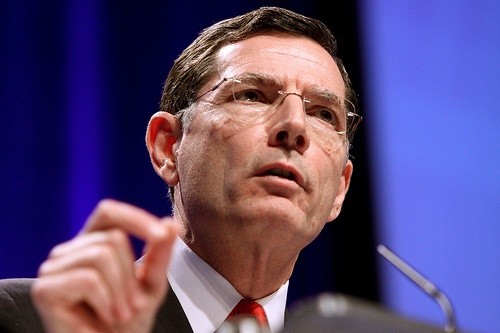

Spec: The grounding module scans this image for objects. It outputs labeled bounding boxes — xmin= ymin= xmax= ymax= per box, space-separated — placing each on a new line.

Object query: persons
xmin=0 ymin=6 xmax=358 ymax=333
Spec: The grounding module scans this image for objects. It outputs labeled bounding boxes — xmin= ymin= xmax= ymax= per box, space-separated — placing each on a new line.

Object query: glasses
xmin=194 ymin=70 xmax=363 ymax=141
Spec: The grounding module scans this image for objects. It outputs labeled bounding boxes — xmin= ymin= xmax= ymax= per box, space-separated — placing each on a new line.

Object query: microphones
xmin=285 ymin=243 xmax=456 ymax=333
